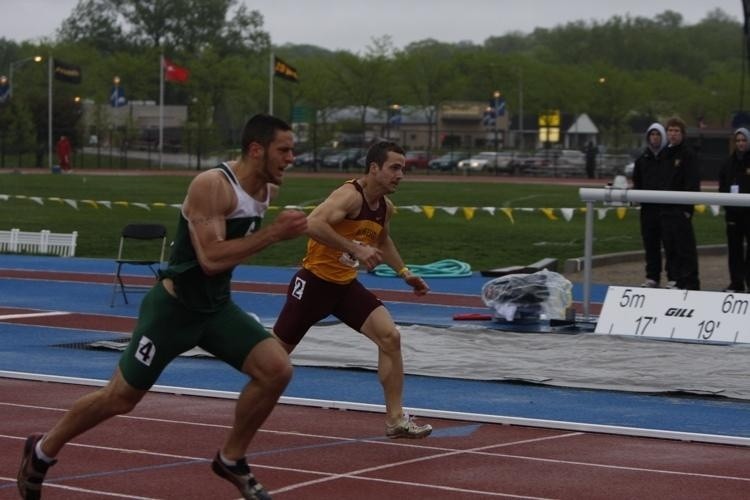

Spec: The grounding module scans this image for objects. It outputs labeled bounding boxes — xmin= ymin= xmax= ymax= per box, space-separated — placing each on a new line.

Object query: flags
xmin=390 ymin=105 xmax=404 ymax=124
xmin=163 ymin=55 xmax=190 ymax=84
xmin=273 ymin=54 xmax=300 ymax=85
xmin=109 ymin=84 xmax=128 ymax=108
xmin=51 ymin=61 xmax=82 ymax=85
xmin=482 ymin=95 xmax=507 ymax=126
xmin=1 ymin=76 xmax=13 ymax=106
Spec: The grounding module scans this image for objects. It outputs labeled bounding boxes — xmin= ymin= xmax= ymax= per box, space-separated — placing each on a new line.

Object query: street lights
xmin=6 ymin=53 xmax=42 ymax=98
xmin=113 ymin=75 xmax=121 ymax=131
xmin=493 ymin=90 xmax=502 ymax=151
xmin=385 ymin=96 xmax=400 ymax=140
xmin=0 ymin=75 xmax=7 ymax=85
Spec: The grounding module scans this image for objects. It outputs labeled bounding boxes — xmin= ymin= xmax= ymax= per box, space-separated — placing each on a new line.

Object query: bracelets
xmin=396 ymin=267 xmax=410 ymax=277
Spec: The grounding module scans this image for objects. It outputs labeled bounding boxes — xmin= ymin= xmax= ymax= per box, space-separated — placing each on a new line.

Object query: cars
xmin=84 ymin=124 xmax=193 ymax=153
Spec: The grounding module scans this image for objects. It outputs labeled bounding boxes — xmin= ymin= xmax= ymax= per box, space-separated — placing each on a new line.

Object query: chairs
xmin=109 ymin=223 xmax=168 ymax=311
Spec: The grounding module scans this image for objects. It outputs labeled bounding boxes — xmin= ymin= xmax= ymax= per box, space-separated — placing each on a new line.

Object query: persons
xmin=718 ymin=127 xmax=750 ymax=292
xmin=656 ymin=115 xmax=703 ymax=291
xmin=582 ymin=138 xmax=600 ymax=178
xmin=55 ymin=132 xmax=73 ymax=170
xmin=268 ymin=140 xmax=433 ymax=440
xmin=631 ymin=120 xmax=671 ymax=287
xmin=17 ymin=114 xmax=308 ymax=500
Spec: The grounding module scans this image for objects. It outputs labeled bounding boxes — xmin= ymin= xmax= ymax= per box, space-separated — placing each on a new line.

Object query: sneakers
xmin=17 ymin=434 xmax=57 ymax=500
xmin=384 ymin=413 xmax=433 ymax=439
xmin=211 ymin=451 xmax=271 ymax=500
